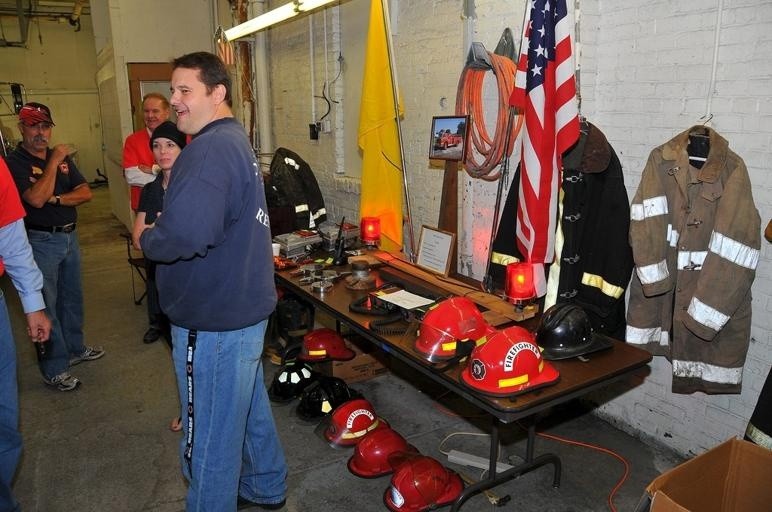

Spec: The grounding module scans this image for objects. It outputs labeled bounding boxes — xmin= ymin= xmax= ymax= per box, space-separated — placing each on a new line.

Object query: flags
xmin=357 ymin=1 xmax=407 ymax=254
xmin=508 ymin=1 xmax=582 ymax=298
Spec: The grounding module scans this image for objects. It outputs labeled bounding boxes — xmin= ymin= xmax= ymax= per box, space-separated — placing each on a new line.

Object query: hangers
xmin=572 ymin=93 xmax=591 ymax=137
xmin=685 ymin=112 xmax=717 ymax=163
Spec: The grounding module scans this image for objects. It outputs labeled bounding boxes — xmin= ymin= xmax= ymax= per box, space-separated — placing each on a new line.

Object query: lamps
xmin=358 ymin=216 xmax=380 ymax=251
xmin=506 ymin=260 xmax=538 ymax=309
xmin=221 ymin=0 xmax=349 ymax=43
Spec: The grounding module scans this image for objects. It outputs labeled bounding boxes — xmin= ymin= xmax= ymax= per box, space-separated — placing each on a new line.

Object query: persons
xmin=6 ymin=100 xmax=106 ymax=393
xmin=131 ymin=122 xmax=185 ymax=431
xmin=0 ymin=156 xmax=53 ymax=510
xmin=139 ymin=52 xmax=291 ymax=512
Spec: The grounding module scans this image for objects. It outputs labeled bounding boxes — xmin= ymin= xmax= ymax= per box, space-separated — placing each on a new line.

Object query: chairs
xmin=122 ymin=232 xmax=149 ymax=309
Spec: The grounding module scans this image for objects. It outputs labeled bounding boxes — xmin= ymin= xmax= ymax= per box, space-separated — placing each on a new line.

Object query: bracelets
xmin=55 ymin=191 xmax=60 ymax=207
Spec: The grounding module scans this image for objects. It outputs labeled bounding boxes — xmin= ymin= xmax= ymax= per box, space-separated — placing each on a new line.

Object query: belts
xmin=27 ymin=222 xmax=77 ymax=233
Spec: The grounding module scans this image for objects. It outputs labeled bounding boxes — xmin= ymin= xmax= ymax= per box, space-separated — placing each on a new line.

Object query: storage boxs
xmin=640 ymin=433 xmax=772 ymax=512
xmin=312 ymin=350 xmax=390 ymax=388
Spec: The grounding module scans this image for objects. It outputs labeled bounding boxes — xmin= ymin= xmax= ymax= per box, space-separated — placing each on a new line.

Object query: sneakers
xmin=237 ymin=493 xmax=286 ymax=510
xmin=66 ymin=342 xmax=106 ymax=367
xmin=41 ymin=371 xmax=80 ymax=393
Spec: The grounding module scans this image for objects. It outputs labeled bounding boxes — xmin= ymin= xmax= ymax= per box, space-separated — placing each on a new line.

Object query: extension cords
xmin=447 ymin=449 xmax=521 ymax=480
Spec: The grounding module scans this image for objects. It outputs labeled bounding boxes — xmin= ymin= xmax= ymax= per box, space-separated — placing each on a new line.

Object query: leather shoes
xmin=142 ymin=328 xmax=164 ymax=344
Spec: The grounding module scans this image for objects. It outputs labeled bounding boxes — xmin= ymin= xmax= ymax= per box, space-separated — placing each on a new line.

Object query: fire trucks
xmin=434 ymin=132 xmax=464 ymax=152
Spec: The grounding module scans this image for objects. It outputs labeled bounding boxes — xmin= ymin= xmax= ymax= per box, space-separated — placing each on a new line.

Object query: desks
xmin=271 ymin=231 xmax=653 ymax=512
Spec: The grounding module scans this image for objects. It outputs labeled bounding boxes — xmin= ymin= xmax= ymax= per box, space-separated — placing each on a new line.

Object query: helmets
xmin=458 ymin=325 xmax=561 ymax=400
xmin=383 ymin=455 xmax=465 ymax=512
xmin=295 ymin=326 xmax=356 ymax=363
xmin=295 ymin=376 xmax=366 ymax=422
xmin=323 ymin=399 xmax=391 ymax=449
xmin=267 ymin=359 xmax=322 ymax=404
xmin=346 ymin=426 xmax=424 ymax=479
xmin=526 ymin=301 xmax=615 ymax=362
xmin=413 ymin=296 xmax=497 ymax=361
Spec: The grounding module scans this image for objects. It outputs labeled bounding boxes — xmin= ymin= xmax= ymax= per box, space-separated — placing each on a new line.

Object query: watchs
xmin=121 ymin=92 xmax=192 ymax=343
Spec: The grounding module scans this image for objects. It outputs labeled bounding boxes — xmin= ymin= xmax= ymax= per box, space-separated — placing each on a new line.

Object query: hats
xmin=149 ymin=121 xmax=187 ymax=152
xmin=19 ymin=102 xmax=56 ymax=128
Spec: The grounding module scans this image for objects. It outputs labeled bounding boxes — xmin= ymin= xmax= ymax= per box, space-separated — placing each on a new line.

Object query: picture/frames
xmin=427 ymin=115 xmax=474 ymax=163
xmin=413 ymin=224 xmax=458 ymax=279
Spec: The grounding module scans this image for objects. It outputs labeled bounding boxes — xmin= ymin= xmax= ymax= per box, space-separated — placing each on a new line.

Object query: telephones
xmin=333 ymin=217 xmax=347 ymax=266
xmin=370 ymin=277 xmax=408 ymax=312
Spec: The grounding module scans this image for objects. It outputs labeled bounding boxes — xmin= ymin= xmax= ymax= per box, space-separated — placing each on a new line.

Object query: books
xmin=272 ymin=221 xmax=359 ymax=258
